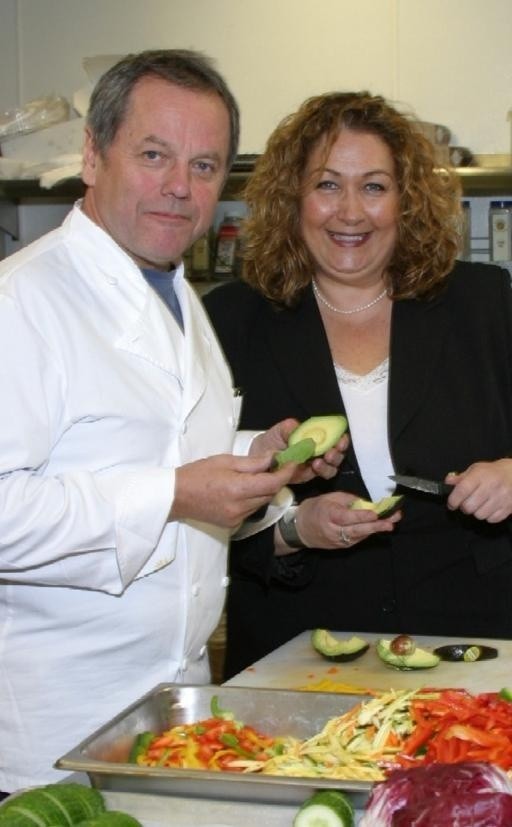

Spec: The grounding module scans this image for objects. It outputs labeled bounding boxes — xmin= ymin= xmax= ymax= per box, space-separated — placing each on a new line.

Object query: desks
xmin=0 ymin=627 xmax=512 ymax=827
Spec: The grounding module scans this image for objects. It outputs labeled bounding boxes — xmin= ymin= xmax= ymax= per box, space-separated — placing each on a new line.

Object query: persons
xmin=2 ymin=48 xmax=352 ymax=808
xmin=201 ymin=89 xmax=511 ymax=681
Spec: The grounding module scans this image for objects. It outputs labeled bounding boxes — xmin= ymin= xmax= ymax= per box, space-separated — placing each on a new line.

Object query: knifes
xmin=387 ymin=472 xmax=456 ymax=498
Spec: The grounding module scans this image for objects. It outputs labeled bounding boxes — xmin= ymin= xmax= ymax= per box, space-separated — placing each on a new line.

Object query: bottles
xmin=191 ymin=226 xmax=215 ymax=280
xmin=486 ymin=199 xmax=511 ymax=263
xmin=454 ymin=199 xmax=471 ymax=262
xmin=212 ymin=209 xmax=246 ymax=280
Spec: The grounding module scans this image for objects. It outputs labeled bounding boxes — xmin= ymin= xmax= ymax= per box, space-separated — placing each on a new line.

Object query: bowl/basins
xmin=409 ymin=120 xmax=472 ymax=166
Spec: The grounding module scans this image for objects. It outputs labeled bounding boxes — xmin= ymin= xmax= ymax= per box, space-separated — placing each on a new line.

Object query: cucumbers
xmin=1 ymin=784 xmax=141 ymax=826
xmin=294 ymin=789 xmax=356 ymax=827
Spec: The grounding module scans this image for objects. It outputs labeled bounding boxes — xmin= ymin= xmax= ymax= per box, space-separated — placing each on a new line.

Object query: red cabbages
xmin=358 ymin=760 xmax=512 ymax=826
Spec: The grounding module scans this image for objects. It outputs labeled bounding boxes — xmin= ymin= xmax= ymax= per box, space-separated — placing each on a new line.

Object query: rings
xmin=342 ymin=529 xmax=355 ymax=544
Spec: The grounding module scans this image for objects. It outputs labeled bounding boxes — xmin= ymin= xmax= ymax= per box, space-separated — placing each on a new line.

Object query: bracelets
xmin=277 ymin=506 xmax=310 ymax=552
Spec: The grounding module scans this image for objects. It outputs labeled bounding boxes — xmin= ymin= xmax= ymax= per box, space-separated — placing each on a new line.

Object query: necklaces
xmin=312 ymin=270 xmax=387 ymax=314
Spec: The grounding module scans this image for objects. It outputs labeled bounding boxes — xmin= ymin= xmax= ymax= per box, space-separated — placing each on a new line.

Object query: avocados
xmin=348 ymin=495 xmax=405 ymax=519
xmin=313 ymin=627 xmax=370 ymax=661
xmin=376 ymin=635 xmax=496 ymax=670
xmin=271 ymin=414 xmax=348 ymax=471
